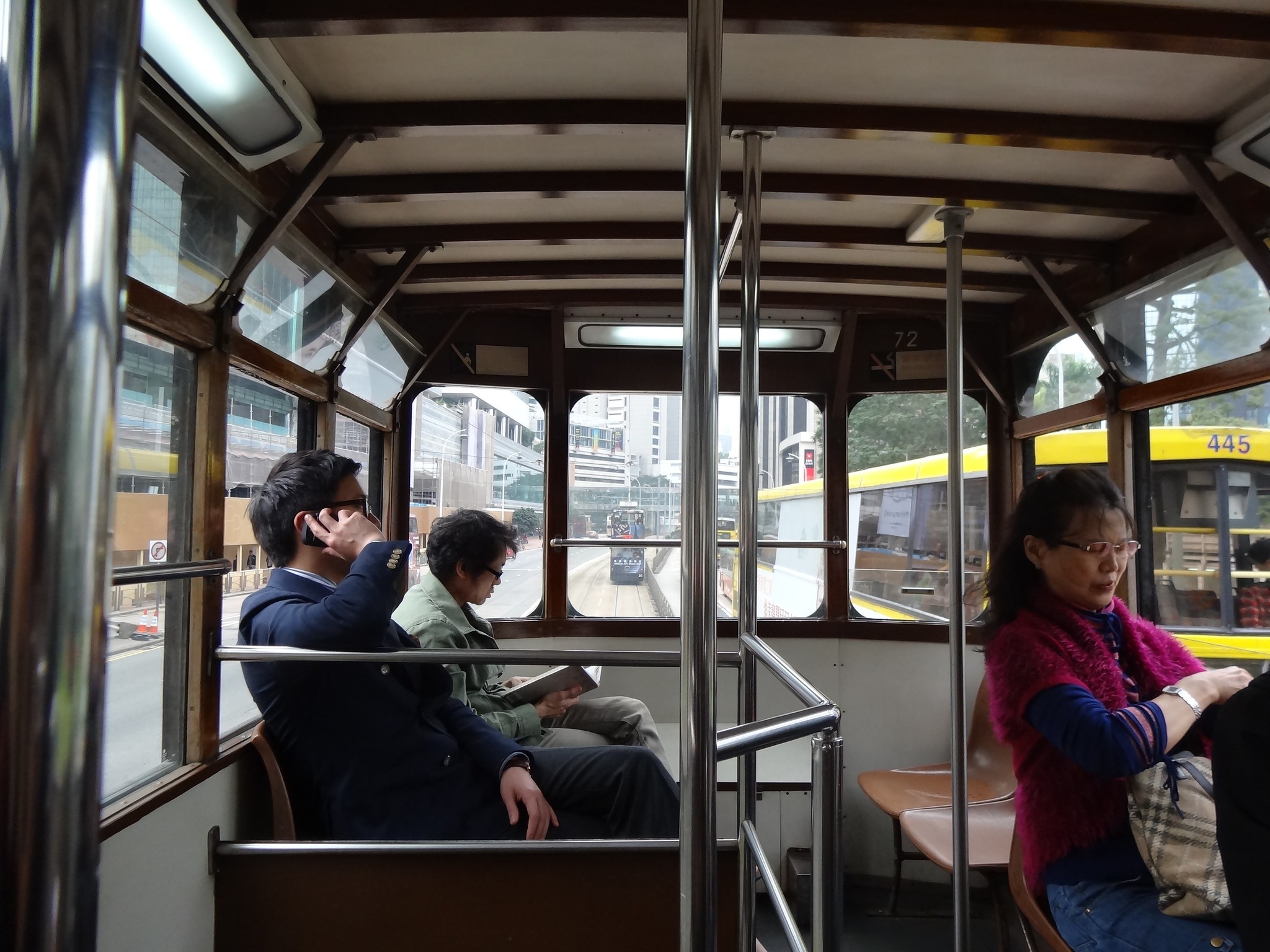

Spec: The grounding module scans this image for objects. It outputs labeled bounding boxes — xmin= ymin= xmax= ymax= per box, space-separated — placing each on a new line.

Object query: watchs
xmin=1161 ymin=685 xmax=1202 ymax=720
xmin=503 ymin=759 xmax=533 ymax=777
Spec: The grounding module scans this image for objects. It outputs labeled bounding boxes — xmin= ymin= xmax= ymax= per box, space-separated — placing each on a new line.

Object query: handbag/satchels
xmin=1124 ymin=757 xmax=1234 ymax=916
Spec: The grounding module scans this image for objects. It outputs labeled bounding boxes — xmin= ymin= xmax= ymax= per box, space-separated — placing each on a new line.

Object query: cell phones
xmin=301 ymin=513 xmax=339 ymax=548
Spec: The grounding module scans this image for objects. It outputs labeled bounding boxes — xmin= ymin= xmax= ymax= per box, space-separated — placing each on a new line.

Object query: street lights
xmin=785 ymin=453 xmax=808 ymax=483
xmin=502 ymin=451 xmax=523 ymax=525
xmin=642 ymin=483 xmax=653 ymax=535
xmin=439 ymin=429 xmax=469 ymax=519
xmin=758 ymin=470 xmax=774 ymax=489
xmin=611 ymin=465 xmax=630 ymax=524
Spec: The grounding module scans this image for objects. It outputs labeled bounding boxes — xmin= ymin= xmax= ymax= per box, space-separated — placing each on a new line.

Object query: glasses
xmin=307 ymin=499 xmax=370 ymax=520
xmin=1035 ymin=535 xmax=1142 ymax=557
xmin=479 ymin=564 xmax=503 ymax=580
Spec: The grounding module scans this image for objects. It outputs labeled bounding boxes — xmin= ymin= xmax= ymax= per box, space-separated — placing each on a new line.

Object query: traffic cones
xmin=132 ymin=608 xmax=150 ymax=641
xmin=148 ymin=610 xmax=161 ymax=639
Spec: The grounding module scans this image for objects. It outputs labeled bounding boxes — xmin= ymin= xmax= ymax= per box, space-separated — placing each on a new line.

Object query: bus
xmin=717 ymin=428 xmax=1270 ymax=677
xmin=607 ymin=546 xmax=644 ymax=585
xmin=606 ymin=508 xmax=644 ymax=540
xmin=409 ymin=513 xmax=421 ymax=588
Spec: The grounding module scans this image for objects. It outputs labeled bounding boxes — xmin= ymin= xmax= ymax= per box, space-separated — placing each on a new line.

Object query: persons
xmin=389 ymin=510 xmax=669 ymax=771
xmin=523 ymin=534 xmax=528 ymax=544
xmin=1247 ymin=537 xmax=1270 ymax=572
xmin=986 ymin=466 xmax=1255 ymax=952
xmin=245 ymin=550 xmax=256 ymax=569
xmin=233 ymin=555 xmax=237 ymax=571
xmin=266 ymin=556 xmax=272 ymax=568
xmin=1211 ymin=671 xmax=1270 ymax=952
xmin=538 ymin=531 xmax=542 ymax=540
xmin=237 ymin=448 xmax=680 ymax=839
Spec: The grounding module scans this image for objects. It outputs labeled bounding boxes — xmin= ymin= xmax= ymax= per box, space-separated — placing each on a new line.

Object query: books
xmin=497 ymin=666 xmax=603 ymax=708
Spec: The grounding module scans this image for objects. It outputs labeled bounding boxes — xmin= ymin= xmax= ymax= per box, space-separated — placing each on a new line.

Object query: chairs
xmin=762 ymin=531 xmax=988 ymax=620
xmin=858 ymin=667 xmax=1019 ymax=915
xmin=900 ymin=798 xmax=1021 ymax=952
xmin=1007 ymin=806 xmax=1073 ymax=952
xmin=250 ymin=718 xmax=295 ymax=842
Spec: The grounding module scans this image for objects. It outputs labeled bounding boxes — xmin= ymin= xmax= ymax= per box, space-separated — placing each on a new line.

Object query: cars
xmin=506 ymin=544 xmax=516 ymax=559
xmin=588 ymin=532 xmax=598 ymax=539
